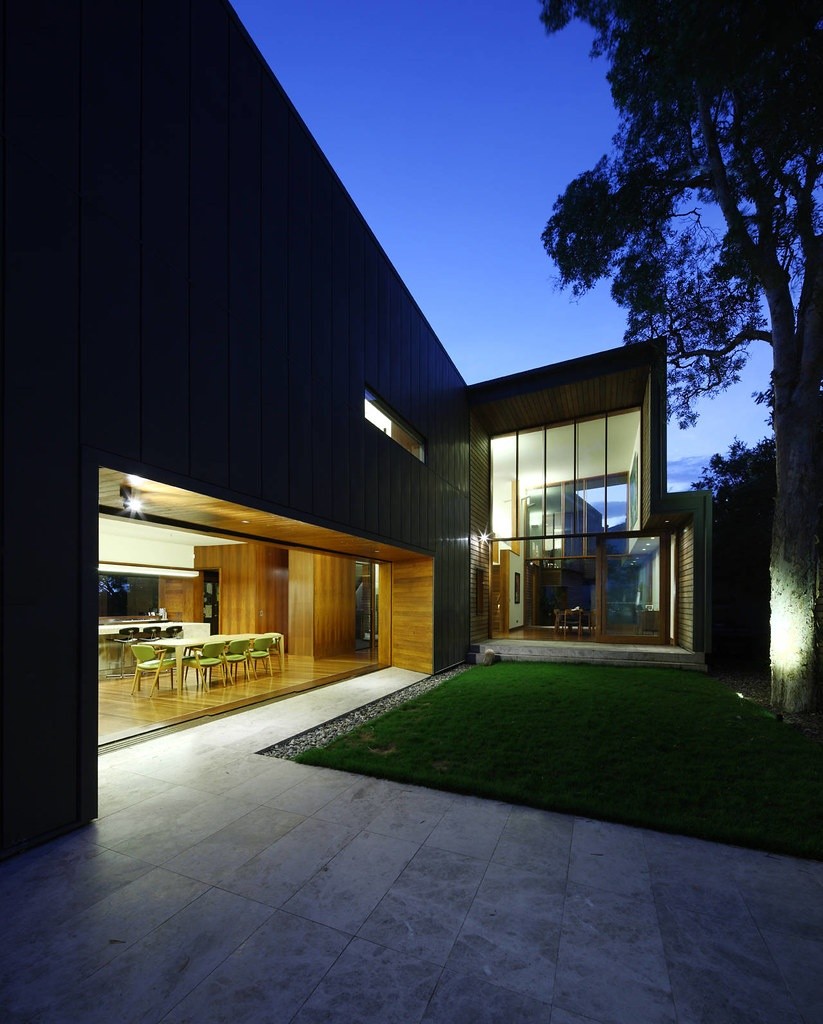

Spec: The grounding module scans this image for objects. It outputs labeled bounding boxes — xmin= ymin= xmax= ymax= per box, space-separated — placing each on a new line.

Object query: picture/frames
xmin=515 ymin=572 xmax=520 ymax=603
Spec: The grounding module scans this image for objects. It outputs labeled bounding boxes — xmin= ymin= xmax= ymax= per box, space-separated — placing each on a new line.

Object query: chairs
xmin=552 ymin=608 xmax=595 ymax=640
xmin=131 ymin=626 xmax=281 ymax=698
xmin=634 ymin=609 xmax=661 ymax=634
xmin=106 ymin=627 xmax=139 ymax=679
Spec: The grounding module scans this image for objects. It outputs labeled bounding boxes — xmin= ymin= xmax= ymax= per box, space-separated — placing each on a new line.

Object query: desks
xmin=138 ymin=634 xmax=284 ymax=695
xmin=560 ymin=611 xmax=591 ymax=633
